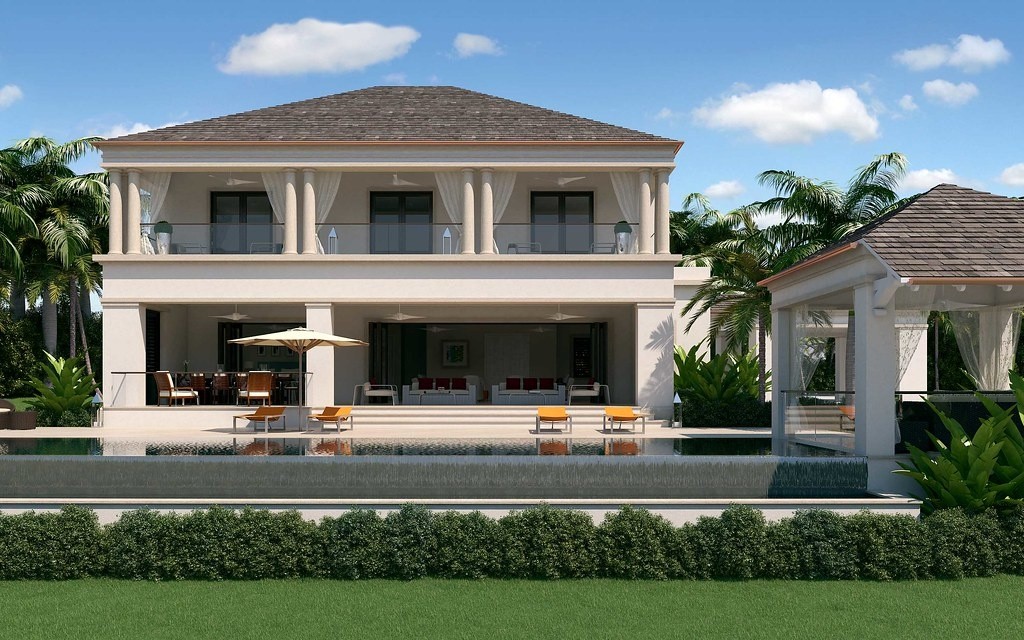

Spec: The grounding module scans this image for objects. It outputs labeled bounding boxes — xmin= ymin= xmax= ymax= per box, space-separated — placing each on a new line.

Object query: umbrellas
xmin=227 ymin=326 xmax=370 ymax=432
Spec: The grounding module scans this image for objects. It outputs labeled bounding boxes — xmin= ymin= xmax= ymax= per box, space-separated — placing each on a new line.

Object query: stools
xmin=11 ymin=411 xmax=38 ymax=430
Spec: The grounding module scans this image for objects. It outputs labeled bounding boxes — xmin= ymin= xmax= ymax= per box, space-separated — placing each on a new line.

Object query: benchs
xmin=590 ymin=242 xmax=617 ymax=253
xmin=506 ymin=242 xmax=542 ymax=254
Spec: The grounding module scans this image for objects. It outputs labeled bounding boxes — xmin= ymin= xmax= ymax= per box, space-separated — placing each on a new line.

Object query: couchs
xmin=894 ymin=390 xmax=1024 ymax=454
xmin=402 ymin=377 xmax=476 ymax=405
xmin=491 ymin=377 xmax=565 ymax=404
xmin=463 ymin=375 xmax=482 ymax=401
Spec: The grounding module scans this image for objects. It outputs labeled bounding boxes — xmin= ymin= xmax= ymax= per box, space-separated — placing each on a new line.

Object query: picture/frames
xmin=256 ymin=346 xmax=266 ymax=357
xmin=441 ymin=339 xmax=469 ymax=369
xmin=271 ymin=346 xmax=280 ymax=357
xmin=285 ymin=346 xmax=294 ymax=357
xmin=571 ymin=334 xmax=593 ymax=380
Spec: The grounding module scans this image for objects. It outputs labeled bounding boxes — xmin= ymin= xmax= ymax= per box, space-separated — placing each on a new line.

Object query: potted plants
xmin=614 ymin=221 xmax=632 ymax=255
xmin=154 ymin=221 xmax=172 ymax=255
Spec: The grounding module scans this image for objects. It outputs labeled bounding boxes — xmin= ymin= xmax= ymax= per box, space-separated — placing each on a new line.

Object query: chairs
xmin=154 ymin=371 xmax=199 ymax=407
xmin=0 ymin=399 xmax=16 ymax=431
xmin=211 ymin=373 xmax=230 ymax=406
xmin=249 ymin=233 xmax=325 ymax=254
xmin=149 ymin=236 xmax=207 ymax=253
xmin=236 ymin=370 xmax=273 ymax=406
xmin=603 ymin=438 xmax=646 ymax=455
xmin=838 ymin=406 xmax=856 ymax=431
xmin=306 ymin=406 xmax=353 ymax=434
xmin=353 ymin=382 xmax=399 ymax=405
xmin=232 ymin=406 xmax=287 ymax=434
xmin=232 ymin=438 xmax=285 ymax=456
xmin=602 ymin=407 xmax=646 ymax=434
xmin=536 ymin=407 xmax=573 ymax=434
xmin=307 ymin=438 xmax=354 ymax=458
xmin=189 ymin=372 xmax=210 ymax=405
xmin=567 ymin=382 xmax=611 ymax=405
xmin=536 ymin=438 xmax=570 ymax=456
xmin=230 ymin=373 xmax=248 ymax=405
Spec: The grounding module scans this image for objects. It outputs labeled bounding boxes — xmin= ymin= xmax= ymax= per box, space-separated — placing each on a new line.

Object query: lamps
xmin=245 ymin=360 xmax=256 ymax=371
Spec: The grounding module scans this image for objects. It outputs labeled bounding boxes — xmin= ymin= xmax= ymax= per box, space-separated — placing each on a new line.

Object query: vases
xmin=184 ymin=366 xmax=188 ymax=372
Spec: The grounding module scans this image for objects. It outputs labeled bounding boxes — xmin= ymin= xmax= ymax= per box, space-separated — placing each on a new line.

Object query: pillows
xmin=523 ymin=378 xmax=538 ymax=390
xmin=452 ymin=378 xmax=466 ymax=390
xmin=418 ymin=378 xmax=433 ymax=390
xmin=506 ymin=378 xmax=520 ymax=390
xmin=435 ymin=378 xmax=450 ymax=390
xmin=539 ymin=378 xmax=554 ymax=389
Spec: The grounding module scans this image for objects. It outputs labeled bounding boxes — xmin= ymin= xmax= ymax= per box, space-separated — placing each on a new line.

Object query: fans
xmin=523 ymin=325 xmax=561 ymax=333
xmin=418 ymin=324 xmax=462 ymax=333
xmin=372 ymin=172 xmax=422 ymax=187
xmin=207 ymin=169 xmax=258 ymax=187
xmin=379 ymin=304 xmax=427 ymax=321
xmin=208 ymin=304 xmax=255 ymax=320
xmin=533 ymin=173 xmax=587 ymax=186
xmin=538 ymin=304 xmax=586 ymax=321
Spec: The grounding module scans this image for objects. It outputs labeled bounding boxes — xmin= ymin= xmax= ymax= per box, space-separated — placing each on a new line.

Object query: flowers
xmin=183 ymin=360 xmax=193 ymax=367
